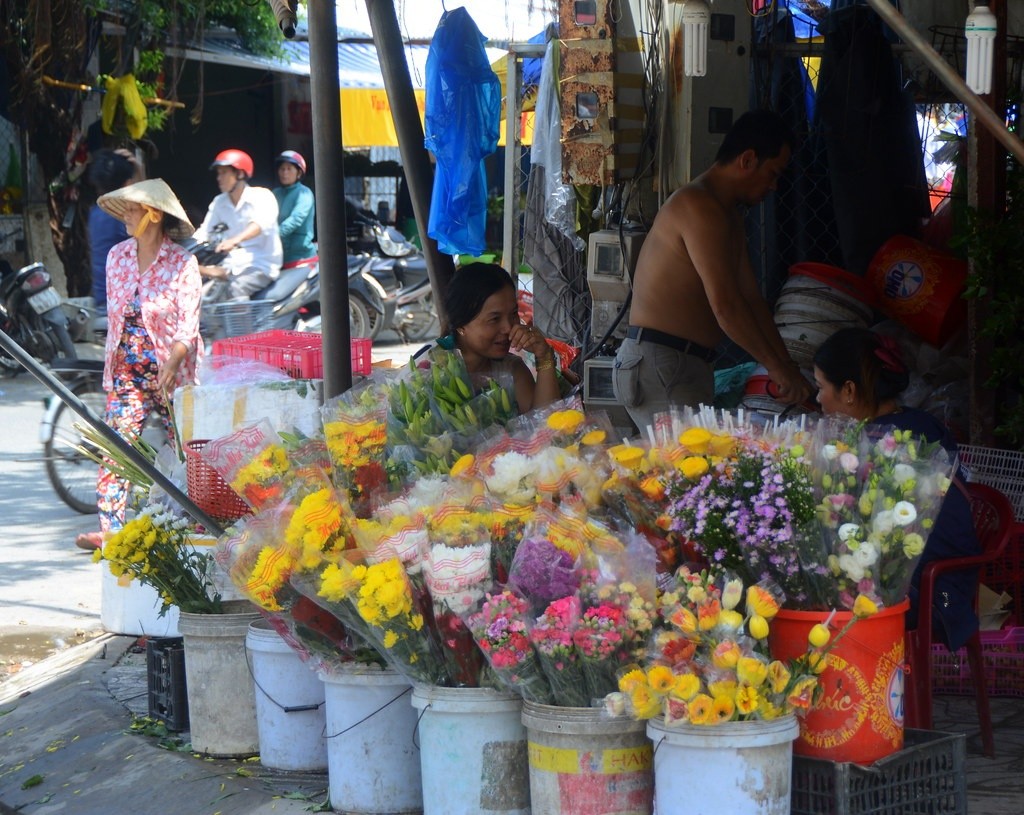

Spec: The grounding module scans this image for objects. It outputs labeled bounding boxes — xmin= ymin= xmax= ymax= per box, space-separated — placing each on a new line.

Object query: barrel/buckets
xmin=767 ymin=593 xmax=910 ymax=767
xmin=867 ymin=234 xmax=971 ymax=347
xmin=176 ymin=599 xmax=799 ymax=815
xmin=736 ymin=261 xmax=878 ymax=434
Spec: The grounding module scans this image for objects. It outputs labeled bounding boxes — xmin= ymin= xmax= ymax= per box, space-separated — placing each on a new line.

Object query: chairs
xmin=903 ymin=482 xmax=1012 ymax=758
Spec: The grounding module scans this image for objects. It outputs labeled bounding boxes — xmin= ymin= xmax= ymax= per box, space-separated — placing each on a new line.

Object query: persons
xmin=269 ymin=150 xmax=318 ymax=262
xmin=613 ymin=111 xmax=812 ymax=446
xmin=191 ymin=149 xmax=285 ymax=338
xmin=73 ymin=176 xmax=201 ymax=554
xmin=811 ymin=328 xmax=978 ymax=650
xmin=400 ymin=263 xmax=565 ymax=427
xmin=80 ymin=138 xmax=150 ymax=313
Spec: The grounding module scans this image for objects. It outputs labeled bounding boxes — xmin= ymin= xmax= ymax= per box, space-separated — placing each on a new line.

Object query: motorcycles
xmin=1 ymin=227 xmax=90 ymax=383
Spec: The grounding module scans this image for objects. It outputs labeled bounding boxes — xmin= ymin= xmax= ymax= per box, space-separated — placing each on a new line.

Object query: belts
xmin=625 ymin=326 xmax=717 ymax=363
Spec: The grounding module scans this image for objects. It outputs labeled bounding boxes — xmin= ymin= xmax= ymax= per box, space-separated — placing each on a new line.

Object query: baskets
xmin=792 ymin=727 xmax=968 ymax=814
xmin=202 ymin=299 xmax=276 ymax=339
xmin=183 ymin=439 xmax=254 ymax=520
xmin=933 ymin=443 xmax=1024 ymax=697
xmin=212 ymin=329 xmax=373 ymax=380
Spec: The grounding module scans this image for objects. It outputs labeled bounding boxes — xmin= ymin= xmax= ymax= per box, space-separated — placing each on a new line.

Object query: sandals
xmin=77 ymin=532 xmax=102 ymax=552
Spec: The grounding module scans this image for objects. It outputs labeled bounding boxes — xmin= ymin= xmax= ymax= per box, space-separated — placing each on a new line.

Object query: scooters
xmin=174 ymin=209 xmax=438 ymax=352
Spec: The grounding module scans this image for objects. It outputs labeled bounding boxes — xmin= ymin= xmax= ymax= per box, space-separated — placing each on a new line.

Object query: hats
xmin=97 ymin=178 xmax=195 ymax=241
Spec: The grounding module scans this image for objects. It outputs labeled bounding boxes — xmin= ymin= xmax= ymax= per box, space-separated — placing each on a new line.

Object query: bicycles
xmin=15 ymin=264 xmax=278 ymax=511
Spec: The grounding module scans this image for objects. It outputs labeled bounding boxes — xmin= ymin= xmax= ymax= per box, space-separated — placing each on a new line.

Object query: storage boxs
xmin=209 ymin=328 xmax=371 ymax=385
xmin=173 ymin=378 xmax=325 ymax=459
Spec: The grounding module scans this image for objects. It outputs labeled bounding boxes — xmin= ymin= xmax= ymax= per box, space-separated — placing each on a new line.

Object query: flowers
xmin=89 ymin=353 xmax=944 ymax=726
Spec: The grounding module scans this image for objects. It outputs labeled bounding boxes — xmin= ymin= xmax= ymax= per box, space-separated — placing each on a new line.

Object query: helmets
xmin=275 ymin=150 xmax=306 ymax=174
xmin=210 ymin=149 xmax=254 ymax=178
